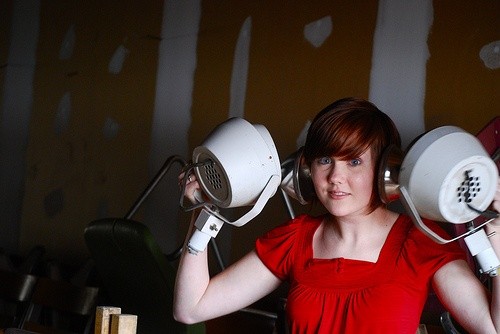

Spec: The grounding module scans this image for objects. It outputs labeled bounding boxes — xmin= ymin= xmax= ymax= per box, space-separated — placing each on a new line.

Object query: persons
xmin=171 ymin=97 xmax=500 ymax=334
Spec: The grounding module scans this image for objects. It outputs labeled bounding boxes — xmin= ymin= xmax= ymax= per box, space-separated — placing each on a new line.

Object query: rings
xmin=188 ymin=176 xmax=192 ymax=182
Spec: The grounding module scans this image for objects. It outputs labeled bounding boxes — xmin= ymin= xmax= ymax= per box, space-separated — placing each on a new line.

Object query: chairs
xmin=80 ymin=217 xmax=206 ymax=334
